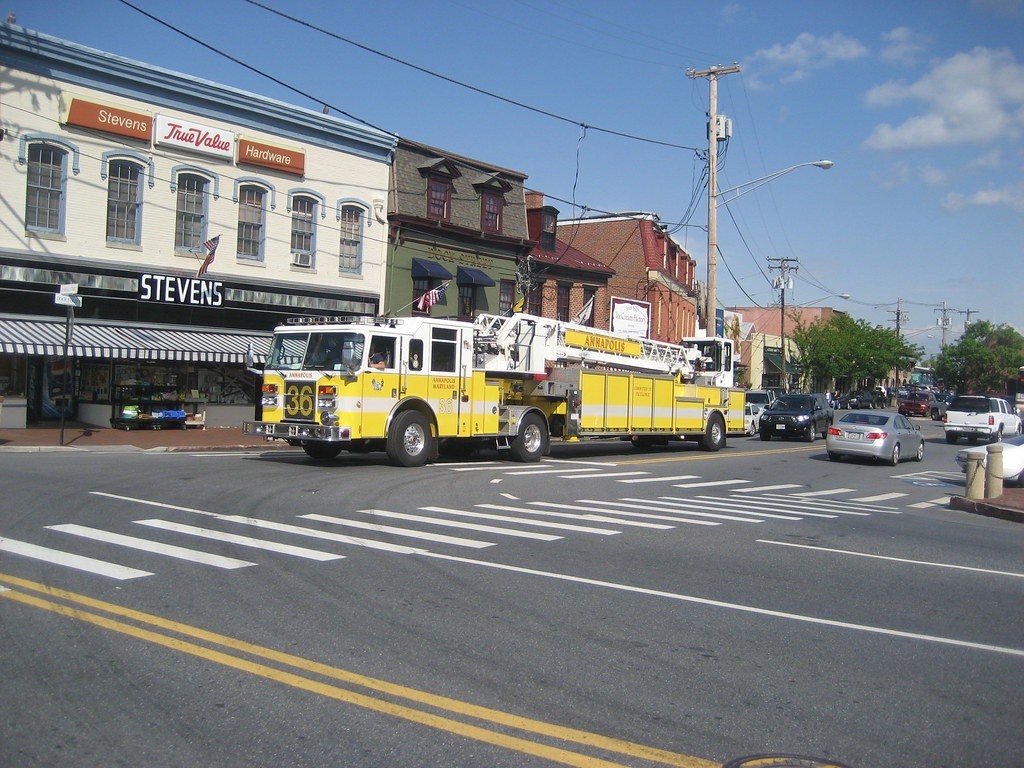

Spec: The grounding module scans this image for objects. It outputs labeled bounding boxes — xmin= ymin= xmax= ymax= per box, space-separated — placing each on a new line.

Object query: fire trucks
xmin=241 ymin=310 xmax=749 ymax=469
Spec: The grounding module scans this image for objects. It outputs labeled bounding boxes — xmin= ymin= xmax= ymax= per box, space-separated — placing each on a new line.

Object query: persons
xmin=368 ymin=342 xmax=386 ymax=368
xmin=726 ymin=315 xmax=741 ymax=355
xmin=824 ymin=389 xmax=831 ymax=404
xmin=833 ymin=391 xmax=840 ymax=410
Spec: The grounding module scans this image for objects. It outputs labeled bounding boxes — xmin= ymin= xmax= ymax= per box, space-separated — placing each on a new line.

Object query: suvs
xmin=758 ymin=391 xmax=834 ymax=442
xmin=744 ymin=385 xmax=786 ymax=411
xmin=942 ymin=394 xmax=1022 ymax=445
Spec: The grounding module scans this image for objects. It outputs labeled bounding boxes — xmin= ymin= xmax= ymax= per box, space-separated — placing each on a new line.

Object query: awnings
xmin=456 ymin=265 xmax=495 ymax=287
xmin=0 ymin=318 xmax=309 ymax=364
xmin=411 ymin=258 xmax=453 ymax=281
xmin=766 ymin=353 xmax=799 ymax=375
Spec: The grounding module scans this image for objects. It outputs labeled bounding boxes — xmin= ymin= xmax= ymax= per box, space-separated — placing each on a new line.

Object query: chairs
xmin=375 ymin=343 xmax=392 ymax=369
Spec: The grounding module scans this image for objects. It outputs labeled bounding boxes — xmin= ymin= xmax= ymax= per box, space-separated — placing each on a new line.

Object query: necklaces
xmin=370 ymin=353 xmax=375 ymax=358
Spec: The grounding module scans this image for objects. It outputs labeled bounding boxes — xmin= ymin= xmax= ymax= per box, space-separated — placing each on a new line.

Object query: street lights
xmin=781 ymin=293 xmax=851 ymax=392
xmin=894 ymin=325 xmax=949 ymax=401
xmin=706 ymin=159 xmax=832 ymax=342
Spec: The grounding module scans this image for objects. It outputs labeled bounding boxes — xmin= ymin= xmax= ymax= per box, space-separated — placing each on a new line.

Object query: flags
xmin=575 ymin=301 xmax=593 ymax=325
xmin=417 ymin=285 xmax=447 ymax=310
xmin=513 ymin=291 xmax=532 ymax=313
xmin=196 ymin=235 xmax=219 ymax=280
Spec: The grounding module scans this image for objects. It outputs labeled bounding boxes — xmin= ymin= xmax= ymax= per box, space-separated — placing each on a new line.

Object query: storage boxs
xmin=155 ymin=410 xmax=186 ymax=418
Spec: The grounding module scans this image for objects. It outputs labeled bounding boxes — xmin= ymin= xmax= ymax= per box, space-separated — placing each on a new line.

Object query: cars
xmin=826 ymin=409 xmax=925 ymax=466
xmin=744 ymin=401 xmax=764 ymax=437
xmin=897 ymin=390 xmax=937 ymax=417
xmin=928 ymin=394 xmax=960 ymax=421
xmin=829 ymin=365 xmax=956 ymax=412
